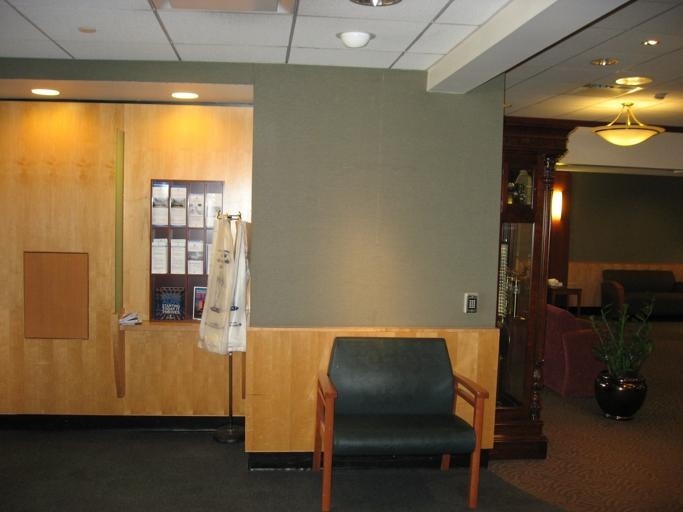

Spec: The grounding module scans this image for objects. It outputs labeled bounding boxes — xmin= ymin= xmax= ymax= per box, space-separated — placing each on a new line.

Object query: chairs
xmin=316 ymin=336 xmax=487 ymax=511
xmin=539 ymin=300 xmax=608 ymax=401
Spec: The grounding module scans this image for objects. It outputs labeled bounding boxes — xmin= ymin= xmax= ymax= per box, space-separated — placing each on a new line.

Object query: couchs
xmin=599 ymin=270 xmax=682 ymax=321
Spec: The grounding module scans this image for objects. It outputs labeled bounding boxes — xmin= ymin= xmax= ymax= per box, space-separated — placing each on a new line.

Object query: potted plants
xmin=589 ymin=306 xmax=655 ymax=424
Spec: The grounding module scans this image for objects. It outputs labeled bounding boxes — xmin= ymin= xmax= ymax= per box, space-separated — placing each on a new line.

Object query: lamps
xmin=592 ymin=105 xmax=660 ymax=147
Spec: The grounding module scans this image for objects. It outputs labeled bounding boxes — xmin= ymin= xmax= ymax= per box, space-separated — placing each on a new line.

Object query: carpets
xmin=0 ymin=427 xmax=561 ymax=512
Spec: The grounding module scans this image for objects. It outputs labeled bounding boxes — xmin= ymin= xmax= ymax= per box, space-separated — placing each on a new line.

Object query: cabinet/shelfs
xmin=488 ymin=123 xmax=571 ymax=463
xmin=149 ymin=177 xmax=223 ymax=324
xmin=546 ymin=283 xmax=581 ymax=314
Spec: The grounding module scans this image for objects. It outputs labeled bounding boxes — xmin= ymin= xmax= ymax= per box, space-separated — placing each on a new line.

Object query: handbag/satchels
xmin=198 ymin=215 xmax=247 ymax=355
